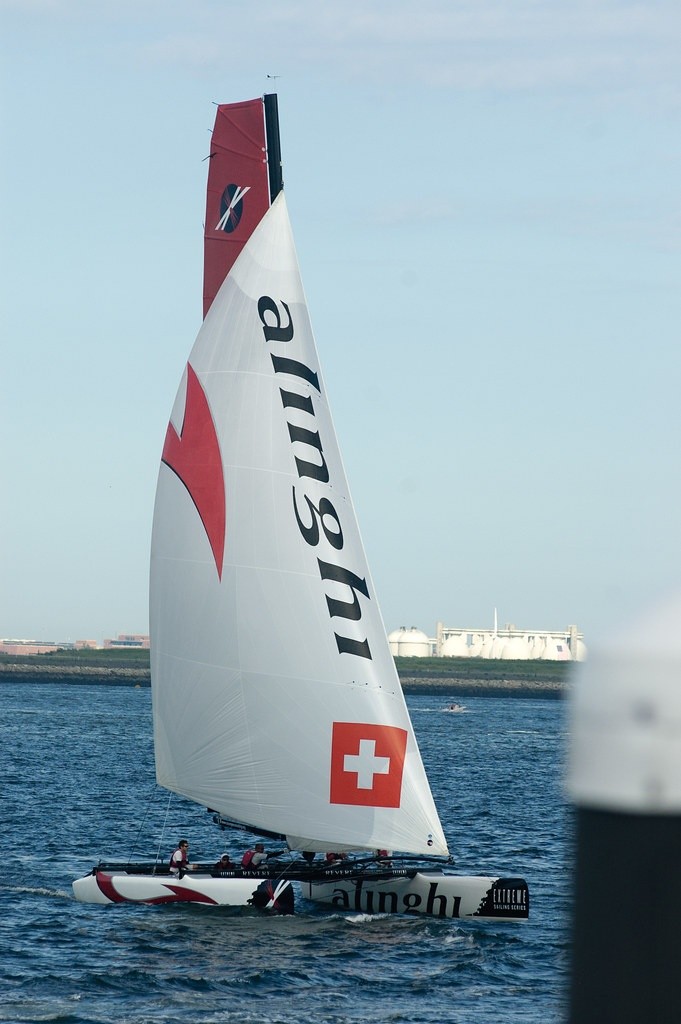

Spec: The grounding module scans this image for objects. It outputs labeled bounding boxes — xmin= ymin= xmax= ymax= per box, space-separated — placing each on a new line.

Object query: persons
xmin=246 ymin=844 xmax=288 ymax=871
xmin=169 ymin=840 xmax=189 ymax=874
xmin=325 ymin=852 xmax=348 ymax=862
xmin=213 ymin=854 xmax=235 ymax=869
xmin=374 ymin=850 xmax=393 ymax=868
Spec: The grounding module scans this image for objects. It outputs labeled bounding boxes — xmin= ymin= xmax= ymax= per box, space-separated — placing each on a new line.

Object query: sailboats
xmin=70 ymin=93 xmax=531 ymax=926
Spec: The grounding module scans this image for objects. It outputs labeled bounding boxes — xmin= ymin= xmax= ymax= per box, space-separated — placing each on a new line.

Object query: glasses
xmin=183 ymin=845 xmax=188 ymax=849
xmin=221 ymin=858 xmax=230 ymax=862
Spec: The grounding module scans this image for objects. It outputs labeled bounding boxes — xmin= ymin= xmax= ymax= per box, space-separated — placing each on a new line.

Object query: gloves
xmin=283 ymin=848 xmax=290 ymax=853
